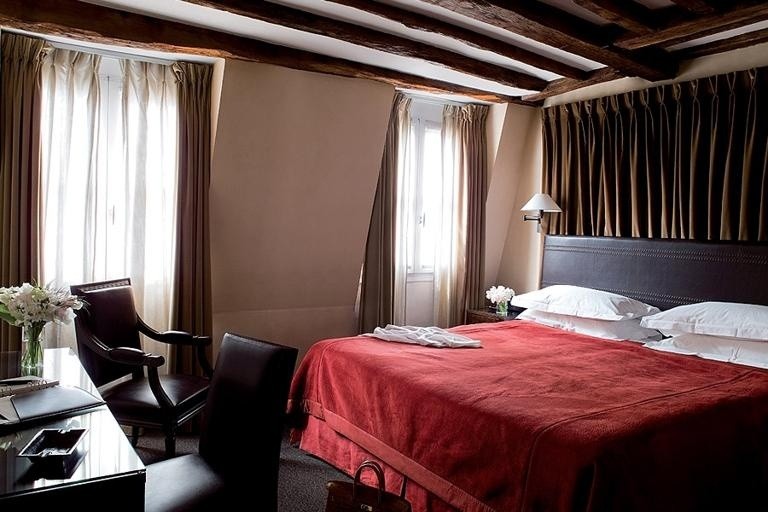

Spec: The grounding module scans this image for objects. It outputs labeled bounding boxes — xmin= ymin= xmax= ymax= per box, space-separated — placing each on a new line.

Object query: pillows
xmin=639 ymin=301 xmax=767 ymax=341
xmin=641 ymin=333 xmax=768 ymax=370
xmin=510 ymin=284 xmax=662 ymax=323
xmin=514 ymin=308 xmax=662 ymax=342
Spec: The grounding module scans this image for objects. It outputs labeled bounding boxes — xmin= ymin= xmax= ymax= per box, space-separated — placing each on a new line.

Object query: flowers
xmin=485 ymin=286 xmax=516 ymax=315
xmin=0 ymin=278 xmax=92 ymax=376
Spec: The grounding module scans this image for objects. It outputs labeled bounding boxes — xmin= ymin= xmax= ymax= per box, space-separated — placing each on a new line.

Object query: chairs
xmin=142 ymin=332 xmax=300 ymax=511
xmin=68 ymin=277 xmax=214 ymax=461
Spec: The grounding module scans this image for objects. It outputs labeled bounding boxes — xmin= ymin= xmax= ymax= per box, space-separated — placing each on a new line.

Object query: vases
xmin=20 ymin=326 xmax=45 ymax=378
xmin=495 ymin=302 xmax=508 ymax=315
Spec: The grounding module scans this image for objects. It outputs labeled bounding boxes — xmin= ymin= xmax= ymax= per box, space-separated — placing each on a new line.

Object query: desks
xmin=0 ymin=347 xmax=148 ymax=512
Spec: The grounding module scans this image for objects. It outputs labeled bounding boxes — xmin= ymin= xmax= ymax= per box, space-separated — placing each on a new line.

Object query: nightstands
xmin=466 ymin=307 xmax=526 ymax=324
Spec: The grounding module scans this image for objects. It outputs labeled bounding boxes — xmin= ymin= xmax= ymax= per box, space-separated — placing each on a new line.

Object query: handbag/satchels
xmin=326 ymin=461 xmax=412 ymax=511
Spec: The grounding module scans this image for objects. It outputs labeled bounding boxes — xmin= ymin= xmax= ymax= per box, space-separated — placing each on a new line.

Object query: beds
xmin=285 ymin=234 xmax=768 ymax=511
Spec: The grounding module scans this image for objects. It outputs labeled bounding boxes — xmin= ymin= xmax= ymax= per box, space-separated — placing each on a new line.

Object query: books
xmin=0 ymin=374 xmax=107 ymax=426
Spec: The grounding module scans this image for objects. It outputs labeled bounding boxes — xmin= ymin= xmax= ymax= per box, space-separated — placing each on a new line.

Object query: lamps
xmin=519 ymin=193 xmax=564 ymax=223
